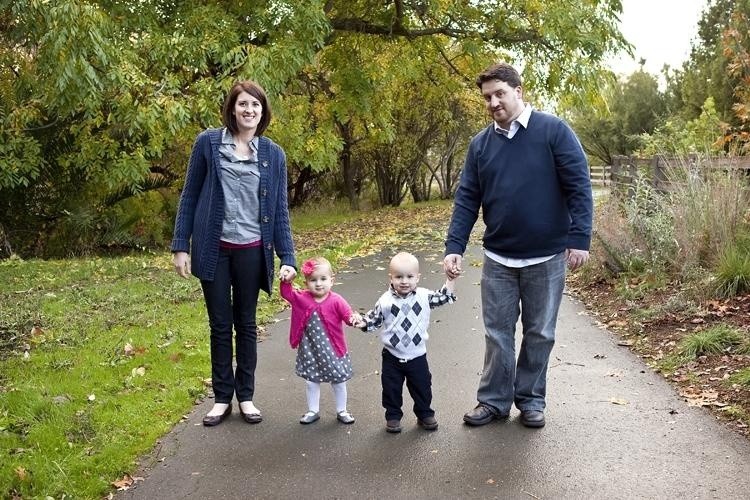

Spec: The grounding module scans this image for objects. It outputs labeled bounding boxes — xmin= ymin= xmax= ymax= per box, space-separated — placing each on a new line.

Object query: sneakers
xmin=386 ymin=419 xmax=402 ymax=432
xmin=418 ymin=416 xmax=438 ymax=429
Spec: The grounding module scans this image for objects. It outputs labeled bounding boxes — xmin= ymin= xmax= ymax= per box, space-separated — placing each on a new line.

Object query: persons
xmin=170 ymin=81 xmax=297 ymax=427
xmin=349 ymin=251 xmax=461 ymax=433
xmin=443 ymin=65 xmax=593 ymax=428
xmin=280 ymin=257 xmax=362 ymax=425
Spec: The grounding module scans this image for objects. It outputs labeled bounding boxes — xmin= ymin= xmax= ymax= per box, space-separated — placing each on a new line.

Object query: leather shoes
xmin=239 ymin=402 xmax=262 ymax=423
xmin=522 ymin=410 xmax=546 ymax=427
xmin=336 ymin=409 xmax=355 ymax=423
xmin=300 ymin=411 xmax=320 ymax=424
xmin=463 ymin=405 xmax=496 ymax=425
xmin=203 ymin=401 xmax=232 ymax=426
